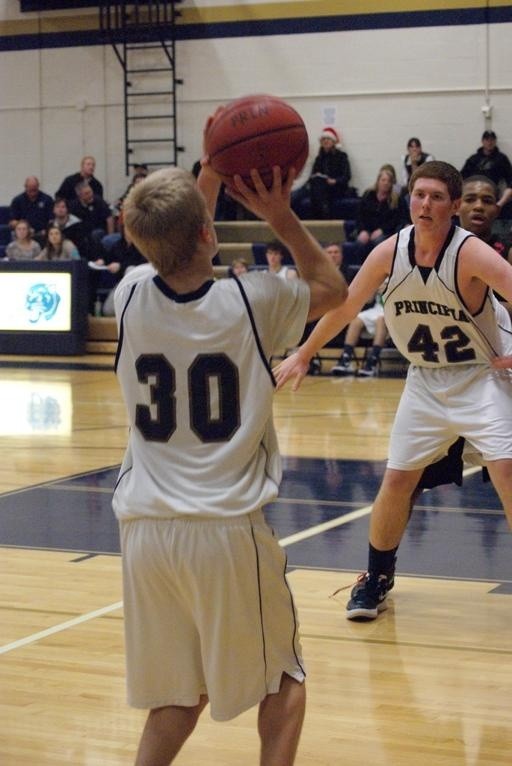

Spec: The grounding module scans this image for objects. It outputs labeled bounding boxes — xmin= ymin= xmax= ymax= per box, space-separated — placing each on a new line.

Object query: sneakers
xmin=351 ymin=558 xmax=397 ymax=598
xmin=357 ymin=355 xmax=379 ymax=376
xmin=346 ymin=572 xmax=388 ymax=620
xmin=332 ymin=352 xmax=356 ymax=373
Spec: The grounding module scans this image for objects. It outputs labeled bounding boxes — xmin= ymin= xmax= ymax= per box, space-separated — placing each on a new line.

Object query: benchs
xmin=1 ymin=192 xmax=512 ymax=354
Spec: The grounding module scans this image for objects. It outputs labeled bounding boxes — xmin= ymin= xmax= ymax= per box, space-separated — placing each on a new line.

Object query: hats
xmin=320 ymin=127 xmax=338 ymax=142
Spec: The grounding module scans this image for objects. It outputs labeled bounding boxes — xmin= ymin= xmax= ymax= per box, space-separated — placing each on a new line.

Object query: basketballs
xmin=206 ymin=96 xmax=307 ymax=190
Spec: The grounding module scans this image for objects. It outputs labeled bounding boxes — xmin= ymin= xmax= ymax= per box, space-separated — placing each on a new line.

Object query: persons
xmin=108 ymin=103 xmax=348 ymax=766
xmin=270 ymin=160 xmax=511 ymax=622
xmin=404 ymin=173 xmax=512 ymax=525
